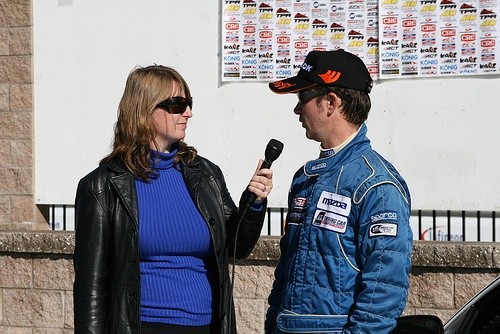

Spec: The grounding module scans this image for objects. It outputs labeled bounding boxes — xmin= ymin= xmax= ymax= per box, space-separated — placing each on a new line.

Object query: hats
xmin=269 ymin=48 xmax=373 ymax=95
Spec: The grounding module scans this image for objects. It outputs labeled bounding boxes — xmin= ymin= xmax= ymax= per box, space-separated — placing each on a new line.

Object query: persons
xmin=73 ymin=62 xmax=274 ymax=334
xmin=264 ymin=48 xmax=413 ymax=334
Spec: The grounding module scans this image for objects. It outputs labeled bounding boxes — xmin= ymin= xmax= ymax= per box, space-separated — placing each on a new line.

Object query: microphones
xmin=247 ymin=139 xmax=284 ymax=207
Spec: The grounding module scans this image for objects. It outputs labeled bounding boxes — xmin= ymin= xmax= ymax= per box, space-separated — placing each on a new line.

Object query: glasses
xmin=156 ymin=96 xmax=193 ymax=114
xmin=296 ymin=90 xmax=329 ymax=105
